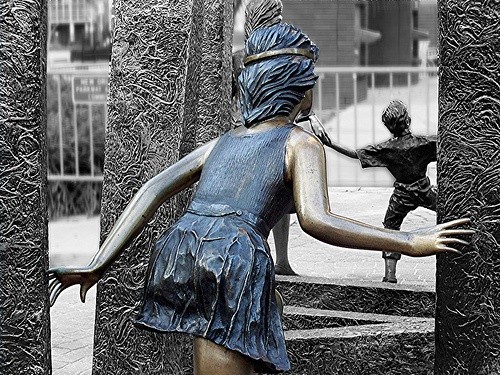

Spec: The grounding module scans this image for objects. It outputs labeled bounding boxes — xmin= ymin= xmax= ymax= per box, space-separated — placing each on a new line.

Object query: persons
xmin=329 ymin=99 xmax=438 ymax=284
xmin=51 ymin=26 xmax=475 ymax=374
xmin=224 ymin=1 xmax=326 ymax=274
